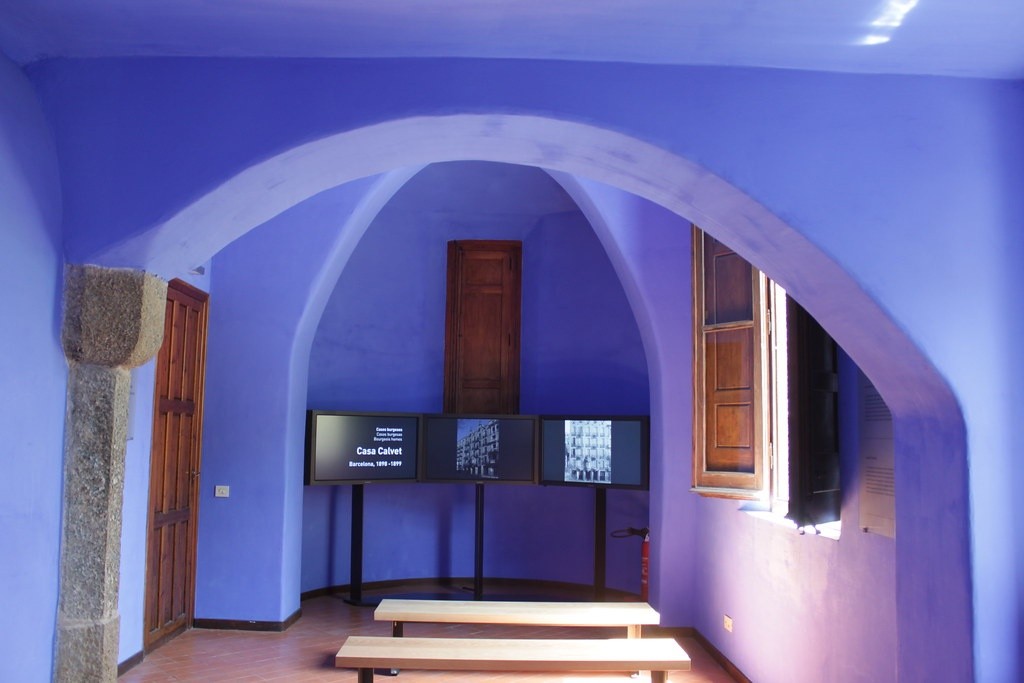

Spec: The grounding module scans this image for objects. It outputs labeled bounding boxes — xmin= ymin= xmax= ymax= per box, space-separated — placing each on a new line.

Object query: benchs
xmin=374 ymin=599 xmax=660 ymax=639
xmin=335 ymin=636 xmax=692 ymax=683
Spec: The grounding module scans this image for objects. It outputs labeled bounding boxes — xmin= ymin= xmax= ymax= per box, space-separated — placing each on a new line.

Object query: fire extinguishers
xmin=609 ymin=526 xmax=649 ymax=602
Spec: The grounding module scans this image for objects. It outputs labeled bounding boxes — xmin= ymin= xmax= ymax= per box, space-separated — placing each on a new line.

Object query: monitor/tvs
xmin=304 ymin=409 xmax=422 ymax=485
xmin=539 ymin=415 xmax=651 ymax=491
xmin=416 ymin=414 xmax=541 ymax=485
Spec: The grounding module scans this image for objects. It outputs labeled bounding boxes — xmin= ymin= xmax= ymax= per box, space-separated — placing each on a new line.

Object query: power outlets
xmin=723 ymin=615 xmax=733 ymax=632
